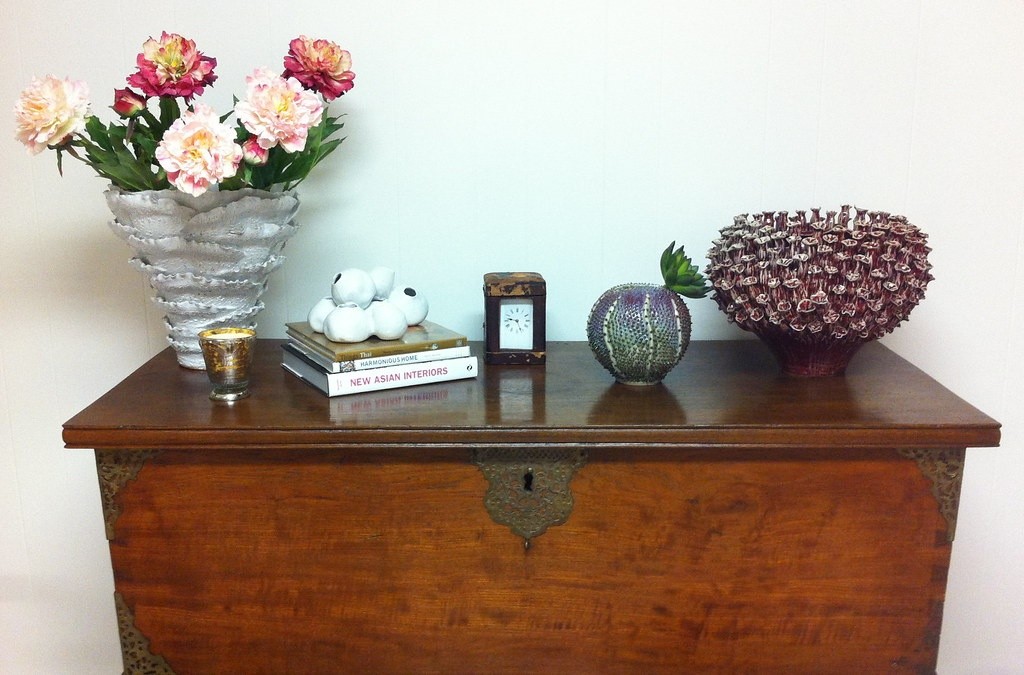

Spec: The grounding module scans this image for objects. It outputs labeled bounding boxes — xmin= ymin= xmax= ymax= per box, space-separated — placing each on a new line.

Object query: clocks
xmin=483 ymin=274 xmax=547 ymax=366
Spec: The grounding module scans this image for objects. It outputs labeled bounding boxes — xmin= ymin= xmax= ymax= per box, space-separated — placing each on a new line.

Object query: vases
xmin=103 ymin=183 xmax=304 ymax=369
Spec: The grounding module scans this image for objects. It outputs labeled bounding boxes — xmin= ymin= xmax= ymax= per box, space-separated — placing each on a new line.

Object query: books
xmin=280 ymin=321 xmax=478 ymax=397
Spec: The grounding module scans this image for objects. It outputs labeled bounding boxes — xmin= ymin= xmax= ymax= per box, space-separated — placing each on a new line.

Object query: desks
xmin=62 ymin=338 xmax=1002 ymax=674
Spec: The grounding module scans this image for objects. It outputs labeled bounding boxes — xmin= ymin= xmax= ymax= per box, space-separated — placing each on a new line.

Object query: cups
xmin=198 ymin=328 xmax=258 ymax=401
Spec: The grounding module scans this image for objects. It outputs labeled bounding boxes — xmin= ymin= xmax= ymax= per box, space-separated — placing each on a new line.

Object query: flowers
xmin=13 ymin=29 xmax=356 ymax=199
